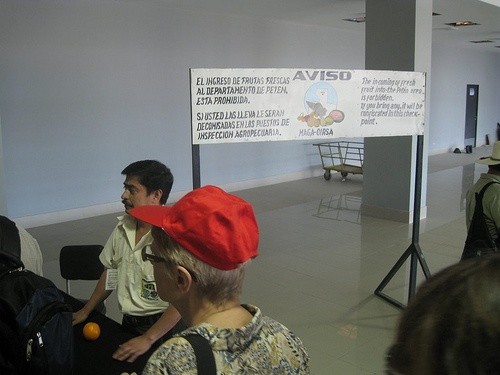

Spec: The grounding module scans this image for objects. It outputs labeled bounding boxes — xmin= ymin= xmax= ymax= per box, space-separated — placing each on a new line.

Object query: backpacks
xmin=0 ymin=215 xmax=25 ymax=281
xmin=0 ymin=267 xmax=76 ymax=375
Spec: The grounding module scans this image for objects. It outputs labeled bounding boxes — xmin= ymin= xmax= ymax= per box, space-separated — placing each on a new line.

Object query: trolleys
xmin=313 ymin=140 xmax=364 ymax=181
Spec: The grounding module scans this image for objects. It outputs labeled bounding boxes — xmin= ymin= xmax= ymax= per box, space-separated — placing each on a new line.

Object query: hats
xmin=126 ymin=186 xmax=259 ymax=270
xmin=476 ymin=140 xmax=500 ymax=166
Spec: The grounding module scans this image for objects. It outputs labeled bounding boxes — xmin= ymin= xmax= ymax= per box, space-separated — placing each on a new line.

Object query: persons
xmin=72 ymin=160 xmax=192 ymax=365
xmin=384 ymin=247 xmax=500 ymax=375
xmin=458 ymin=140 xmax=500 ymax=262
xmin=125 ymin=184 xmax=313 ymax=375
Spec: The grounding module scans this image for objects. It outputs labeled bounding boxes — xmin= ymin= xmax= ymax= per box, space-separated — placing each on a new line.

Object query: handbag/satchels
xmin=458 ymin=192 xmax=498 ymax=267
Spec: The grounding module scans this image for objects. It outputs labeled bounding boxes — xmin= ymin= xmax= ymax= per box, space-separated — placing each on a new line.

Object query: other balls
xmin=83 ymin=323 xmax=100 ymax=339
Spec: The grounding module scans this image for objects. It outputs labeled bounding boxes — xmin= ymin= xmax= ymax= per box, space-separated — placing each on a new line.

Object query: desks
xmin=57 ymin=287 xmax=158 ymax=375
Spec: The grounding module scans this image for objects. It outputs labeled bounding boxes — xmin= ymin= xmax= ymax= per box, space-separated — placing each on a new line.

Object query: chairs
xmin=59 ymin=245 xmax=107 ymax=316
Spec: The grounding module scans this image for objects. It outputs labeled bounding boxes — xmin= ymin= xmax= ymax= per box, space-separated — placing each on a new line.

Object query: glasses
xmin=386 ymin=343 xmax=408 ymax=375
xmin=141 ymin=243 xmax=197 ymax=284
